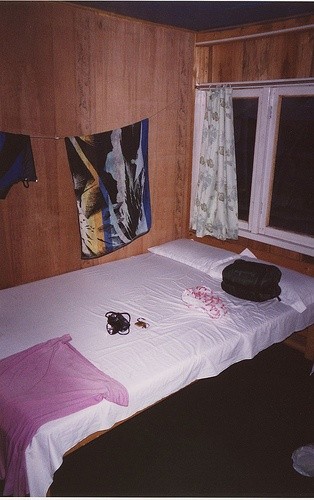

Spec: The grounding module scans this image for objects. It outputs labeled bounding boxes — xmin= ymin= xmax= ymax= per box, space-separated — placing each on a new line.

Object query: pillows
xmin=209 ymin=247 xmax=313 ymax=313
xmin=146 ymin=238 xmax=238 ymax=273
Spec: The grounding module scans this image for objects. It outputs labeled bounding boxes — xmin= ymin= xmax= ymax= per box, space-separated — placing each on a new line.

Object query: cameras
xmin=105 ymin=312 xmax=130 ymax=336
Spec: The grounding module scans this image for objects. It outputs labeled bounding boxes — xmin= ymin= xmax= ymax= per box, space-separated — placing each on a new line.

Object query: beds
xmin=0 ymin=238 xmax=314 ymax=497
